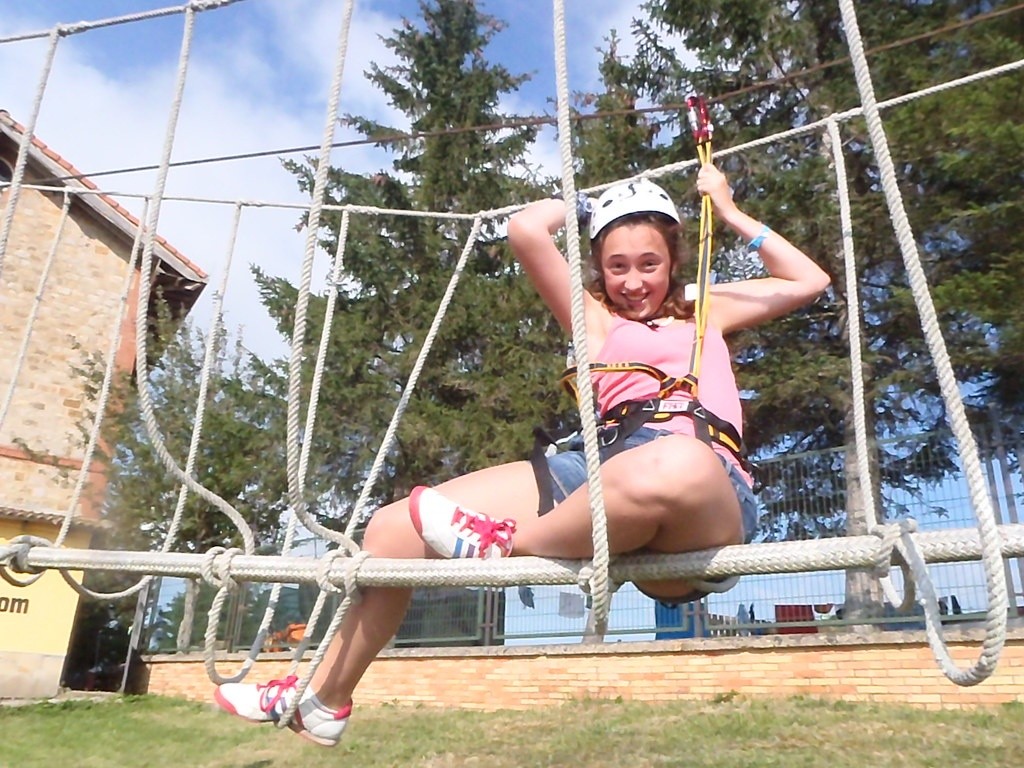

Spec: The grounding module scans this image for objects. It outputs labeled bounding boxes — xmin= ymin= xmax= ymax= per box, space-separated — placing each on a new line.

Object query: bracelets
xmin=747 ymin=225 xmax=772 ymax=253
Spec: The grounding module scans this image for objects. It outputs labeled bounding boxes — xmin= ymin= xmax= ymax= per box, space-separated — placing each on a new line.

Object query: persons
xmin=213 ymin=163 xmax=833 ymax=746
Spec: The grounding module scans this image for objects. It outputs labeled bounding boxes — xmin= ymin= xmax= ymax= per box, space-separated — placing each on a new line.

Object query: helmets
xmin=587 ymin=177 xmax=681 ymax=239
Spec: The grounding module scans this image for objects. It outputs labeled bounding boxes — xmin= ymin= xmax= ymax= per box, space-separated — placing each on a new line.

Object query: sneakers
xmin=214 ymin=674 xmax=353 ymax=747
xmin=410 ymin=484 xmax=517 ymax=558
